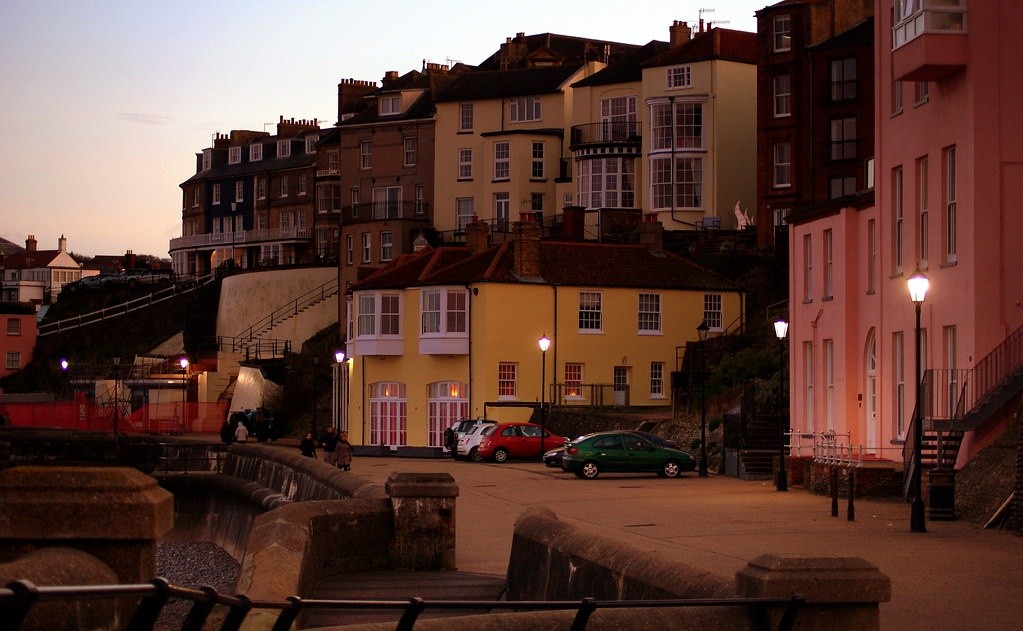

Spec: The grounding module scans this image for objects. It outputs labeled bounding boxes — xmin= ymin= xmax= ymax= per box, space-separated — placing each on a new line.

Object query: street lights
xmin=61 ymin=359 xmax=68 ymax=397
xmin=904 ymin=262 xmax=931 ymax=532
xmin=770 ymin=313 xmax=790 ymax=491
xmin=231 ymin=202 xmax=238 ymax=271
xmin=334 ymin=348 xmax=346 ymax=441
xmin=181 ymin=358 xmax=188 ymax=432
xmin=537 ymin=332 xmax=552 ymax=462
xmin=695 ymin=318 xmax=711 ymax=477
xmin=111 ymin=351 xmax=121 ymax=436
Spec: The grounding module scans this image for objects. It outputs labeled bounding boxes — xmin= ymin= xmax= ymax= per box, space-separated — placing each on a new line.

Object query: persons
xmin=245 ymin=407 xmax=275 ymax=437
xmin=319 ymin=424 xmax=352 ymax=471
xmin=235 ymin=422 xmax=248 ymax=444
xmin=220 ymin=421 xmax=233 ymax=445
xmin=299 ymin=432 xmax=317 ymax=459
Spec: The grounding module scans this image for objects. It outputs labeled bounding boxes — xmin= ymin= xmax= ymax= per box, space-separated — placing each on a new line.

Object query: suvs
xmin=442 ymin=418 xmax=498 ymax=461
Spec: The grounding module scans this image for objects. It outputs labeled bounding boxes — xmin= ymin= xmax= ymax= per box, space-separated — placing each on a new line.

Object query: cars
xmin=562 ymin=433 xmax=698 ymax=479
xmin=477 ymin=422 xmax=570 ymax=464
xmin=457 ymin=423 xmax=531 ymax=462
xmin=543 ymin=431 xmax=681 ymax=472
